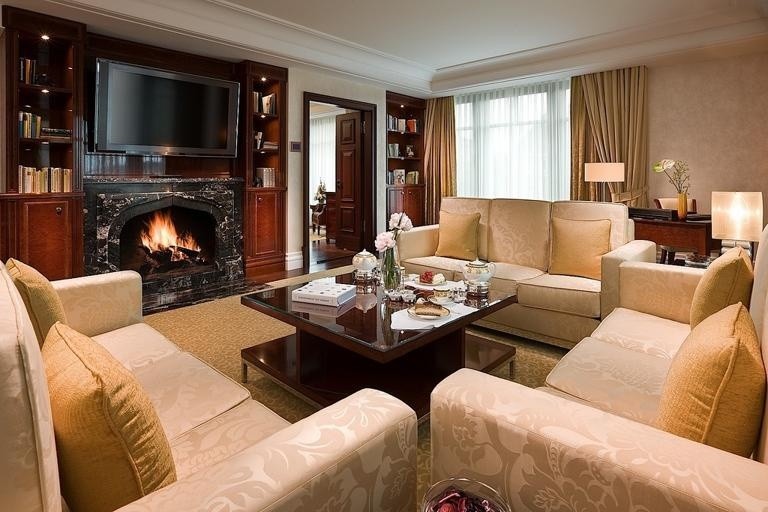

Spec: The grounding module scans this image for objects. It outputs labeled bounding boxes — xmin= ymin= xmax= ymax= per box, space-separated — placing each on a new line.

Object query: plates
xmin=420 ymin=282 xmax=446 ymax=286
xmin=429 ymin=298 xmax=453 ymax=305
xmin=408 ymin=306 xmax=450 ymax=319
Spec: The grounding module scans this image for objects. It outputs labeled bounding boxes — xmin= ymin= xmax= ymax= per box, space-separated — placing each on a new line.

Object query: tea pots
xmin=354 ymin=294 xmax=377 ymax=314
xmin=458 ymin=257 xmax=497 ymax=284
xmin=352 ymin=249 xmax=377 ymax=270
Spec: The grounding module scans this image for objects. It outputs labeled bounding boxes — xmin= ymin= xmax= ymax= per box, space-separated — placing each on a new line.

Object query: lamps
xmin=711 ymin=191 xmax=764 ymax=247
xmin=584 ymin=163 xmax=625 ymax=203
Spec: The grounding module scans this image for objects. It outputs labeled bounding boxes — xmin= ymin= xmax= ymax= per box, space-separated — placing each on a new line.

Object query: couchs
xmin=429 ymin=224 xmax=768 ymax=512
xmin=0 ymin=257 xmax=418 ymax=512
xmin=393 ymin=196 xmax=657 ymax=350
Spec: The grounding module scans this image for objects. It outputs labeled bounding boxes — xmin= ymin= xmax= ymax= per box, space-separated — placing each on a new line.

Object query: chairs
xmin=654 ymin=198 xmax=697 ymax=214
xmin=312 ymin=204 xmax=326 ymax=235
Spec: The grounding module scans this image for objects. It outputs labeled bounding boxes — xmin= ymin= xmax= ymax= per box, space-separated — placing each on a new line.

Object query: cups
xmin=433 ymin=288 xmax=453 ymax=302
xmin=400 ymin=290 xmax=413 ymax=296
xmin=403 ymin=294 xmax=415 ymax=302
xmin=389 ymin=292 xmax=402 ymax=302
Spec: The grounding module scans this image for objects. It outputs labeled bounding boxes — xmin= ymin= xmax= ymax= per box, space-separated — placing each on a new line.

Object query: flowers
xmin=314 ymin=177 xmax=326 ymax=200
xmin=653 ymin=159 xmax=692 ymax=194
xmin=374 ymin=212 xmax=413 ymax=291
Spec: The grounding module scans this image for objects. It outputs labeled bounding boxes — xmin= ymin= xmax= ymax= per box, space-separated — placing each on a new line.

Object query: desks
xmin=631 ymin=216 xmax=720 ymax=260
xmin=241 ymin=273 xmax=517 ymax=429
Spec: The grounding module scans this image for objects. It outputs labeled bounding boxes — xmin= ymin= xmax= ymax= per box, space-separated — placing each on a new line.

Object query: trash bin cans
xmin=420 ymin=475 xmax=511 ymax=512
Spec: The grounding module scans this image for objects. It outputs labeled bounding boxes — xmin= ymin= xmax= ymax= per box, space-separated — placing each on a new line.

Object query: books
xmin=388 ymin=169 xmax=420 ymax=185
xmin=253 ymin=131 xmax=280 ymax=151
xmin=257 ymin=167 xmax=276 ymax=188
xmin=17 ymin=164 xmax=73 ymax=194
xmin=19 ymin=57 xmax=38 ymax=84
xmin=291 ymin=281 xmax=357 ymax=307
xmin=18 ymin=111 xmax=73 ymax=142
xmin=253 ymin=91 xmax=277 ymax=115
xmin=386 ymin=113 xmax=417 ymax=132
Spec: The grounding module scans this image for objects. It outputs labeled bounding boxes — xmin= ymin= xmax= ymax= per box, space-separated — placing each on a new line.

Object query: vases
xmin=678 ymin=194 xmax=687 ymax=221
xmin=382 ymin=246 xmax=401 ymax=291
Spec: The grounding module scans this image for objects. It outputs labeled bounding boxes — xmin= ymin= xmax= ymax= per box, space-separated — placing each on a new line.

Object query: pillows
xmin=435 ymin=210 xmax=481 ymax=261
xmin=549 ymin=217 xmax=612 ymax=281
xmin=659 ymin=245 xmax=766 ymax=458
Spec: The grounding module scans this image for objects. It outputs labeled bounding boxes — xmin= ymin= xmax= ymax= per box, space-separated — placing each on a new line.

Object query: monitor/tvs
xmin=93 ymin=56 xmax=240 ymax=178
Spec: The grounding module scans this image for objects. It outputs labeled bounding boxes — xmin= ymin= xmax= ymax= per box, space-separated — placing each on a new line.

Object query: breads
xmin=415 ymin=302 xmax=442 ymax=317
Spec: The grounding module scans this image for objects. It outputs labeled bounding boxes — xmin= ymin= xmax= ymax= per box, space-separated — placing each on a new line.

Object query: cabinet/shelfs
xmin=324 ymin=192 xmax=336 ymax=244
xmin=231 ymin=60 xmax=288 ymax=287
xmin=386 ymin=91 xmax=426 ymax=231
xmin=0 ymin=5 xmax=87 ymax=281
